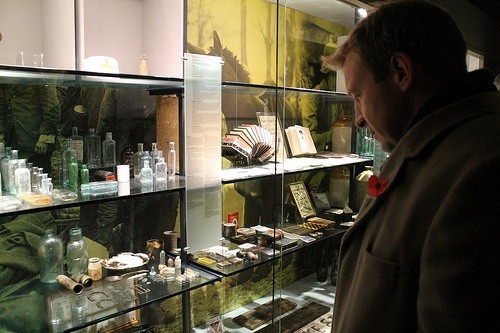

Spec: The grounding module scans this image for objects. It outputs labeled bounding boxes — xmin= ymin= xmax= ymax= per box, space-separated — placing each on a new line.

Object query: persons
xmin=319 ymin=0 xmax=500 ymax=333
xmin=228 ymin=73 xmax=336 ymax=286
xmin=0 ymin=78 xmax=181 ymax=333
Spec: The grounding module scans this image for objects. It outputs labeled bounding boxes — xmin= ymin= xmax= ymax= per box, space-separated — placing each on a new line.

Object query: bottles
xmin=37 ymin=228 xmax=64 ymax=283
xmin=87 ymin=257 xmax=102 ymax=281
xmin=66 ymin=226 xmax=91 ymax=277
xmin=0 ymin=127 xmax=177 ymax=209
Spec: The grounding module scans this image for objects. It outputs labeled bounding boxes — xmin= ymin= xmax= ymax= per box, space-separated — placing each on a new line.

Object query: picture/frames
xmin=256 ymin=112 xmax=290 ymax=159
xmin=287 ymin=181 xmax=316 ymax=220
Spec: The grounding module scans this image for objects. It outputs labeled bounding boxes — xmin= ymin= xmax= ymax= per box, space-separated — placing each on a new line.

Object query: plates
xmin=102 ymin=253 xmax=149 ymax=270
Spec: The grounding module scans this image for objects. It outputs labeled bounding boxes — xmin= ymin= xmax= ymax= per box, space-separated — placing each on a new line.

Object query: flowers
xmin=366 ymin=175 xmax=389 ymax=197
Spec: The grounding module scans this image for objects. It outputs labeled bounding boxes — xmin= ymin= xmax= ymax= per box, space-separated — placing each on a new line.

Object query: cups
xmin=146 ymin=239 xmax=165 ymax=262
xmin=223 ymin=223 xmax=237 ymax=238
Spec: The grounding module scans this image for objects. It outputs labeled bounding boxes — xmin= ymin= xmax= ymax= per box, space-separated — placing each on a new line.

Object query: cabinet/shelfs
xmin=0 ymin=0 xmax=485 ymax=333
